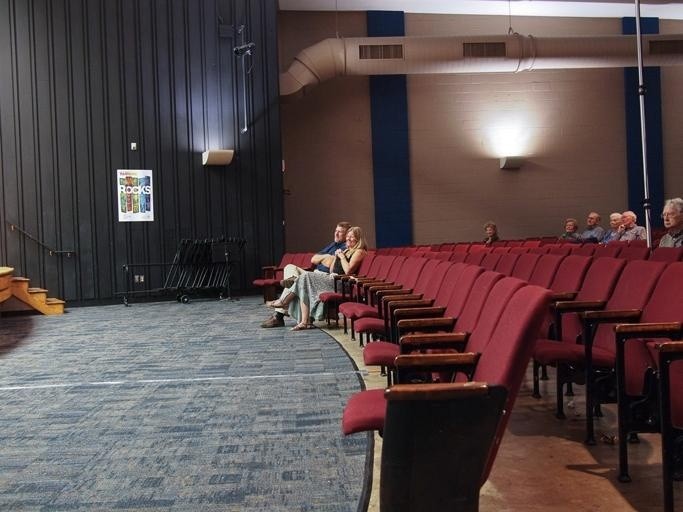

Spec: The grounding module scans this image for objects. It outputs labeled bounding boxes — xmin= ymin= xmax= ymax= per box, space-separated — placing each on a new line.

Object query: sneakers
xmin=280 ymin=276 xmax=297 ymax=288
xmin=260 ymin=315 xmax=285 ymax=328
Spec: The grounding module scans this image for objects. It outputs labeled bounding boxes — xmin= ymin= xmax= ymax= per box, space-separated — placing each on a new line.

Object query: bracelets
xmin=335 ymin=250 xmax=342 ymax=256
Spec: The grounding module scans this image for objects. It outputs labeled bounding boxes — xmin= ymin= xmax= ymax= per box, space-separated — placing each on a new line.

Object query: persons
xmin=558 ymin=219 xmax=580 ymax=243
xmin=257 ymin=221 xmax=350 ymax=328
xmin=612 ymin=208 xmax=647 ymax=243
xmin=600 ymin=211 xmax=621 ymax=246
xmin=479 ymin=221 xmax=499 ymax=245
xmin=581 ymin=211 xmax=605 ymax=243
xmin=656 ymin=198 xmax=683 ymax=249
xmin=266 ymin=225 xmax=367 ymax=335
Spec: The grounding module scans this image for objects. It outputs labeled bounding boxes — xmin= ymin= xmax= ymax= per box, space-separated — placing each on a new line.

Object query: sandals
xmin=266 ymin=300 xmax=289 ymax=311
xmin=289 ymin=321 xmax=312 ymax=331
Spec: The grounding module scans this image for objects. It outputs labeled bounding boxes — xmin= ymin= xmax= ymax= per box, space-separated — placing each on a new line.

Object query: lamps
xmin=202 ymin=150 xmax=235 ymax=165
xmin=499 ymin=156 xmax=524 ymax=170
